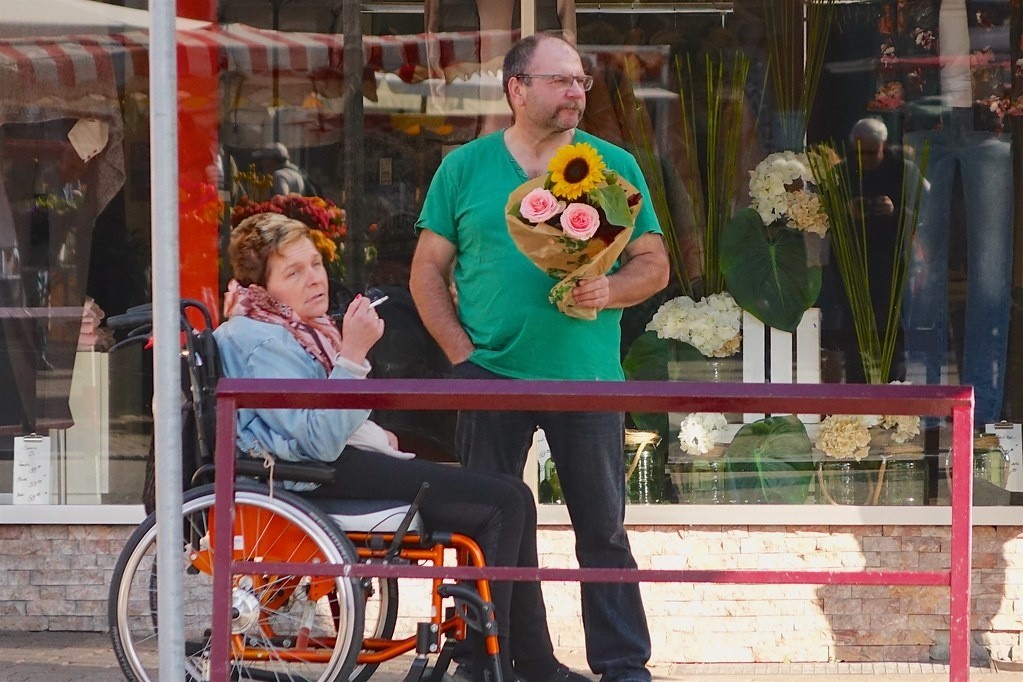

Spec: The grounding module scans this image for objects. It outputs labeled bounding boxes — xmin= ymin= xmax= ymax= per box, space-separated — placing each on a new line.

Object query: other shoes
xmin=514 ymin=658 xmax=586 ymax=682
xmin=451 ymin=654 xmax=513 ymax=682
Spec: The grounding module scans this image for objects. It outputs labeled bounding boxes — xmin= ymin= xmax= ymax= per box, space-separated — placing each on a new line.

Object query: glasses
xmin=517 ymin=74 xmax=594 ymax=97
xmin=857 ymin=142 xmax=884 ymax=156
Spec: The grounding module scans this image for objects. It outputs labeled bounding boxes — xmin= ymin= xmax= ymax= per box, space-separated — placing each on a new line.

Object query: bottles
xmin=946 ymin=433 xmax=1010 ymax=505
xmin=622 ymin=428 xmax=664 ymax=505
xmin=668 ymin=443 xmax=725 ymax=505
xmin=879 ymin=443 xmax=928 ymax=507
xmin=816 ymin=453 xmax=873 ymax=507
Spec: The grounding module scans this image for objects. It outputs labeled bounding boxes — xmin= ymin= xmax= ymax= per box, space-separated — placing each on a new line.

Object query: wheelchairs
xmin=104 ymin=297 xmax=506 ymax=682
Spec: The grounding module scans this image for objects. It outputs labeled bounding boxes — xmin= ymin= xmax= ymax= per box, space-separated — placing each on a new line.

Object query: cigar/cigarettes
xmin=368 ymin=296 xmax=388 ymax=309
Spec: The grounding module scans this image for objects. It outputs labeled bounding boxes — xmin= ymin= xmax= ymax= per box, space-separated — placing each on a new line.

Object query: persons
xmin=409 ymin=34 xmax=669 ymax=682
xmin=921 ymin=100 xmax=1022 ymax=423
xmin=827 ymin=119 xmax=921 ymax=384
xmin=209 ymin=212 xmax=591 ymax=682
xmin=255 ymin=142 xmax=307 ymax=196
xmin=207 ymin=143 xmax=240 ymax=202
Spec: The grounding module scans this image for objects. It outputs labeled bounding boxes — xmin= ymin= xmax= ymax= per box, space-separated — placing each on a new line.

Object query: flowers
xmin=230 ymin=163 xmax=378 ymax=275
xmin=505 ymin=142 xmax=643 ymax=320
xmin=611 ymin=0 xmax=1023 ymax=504
